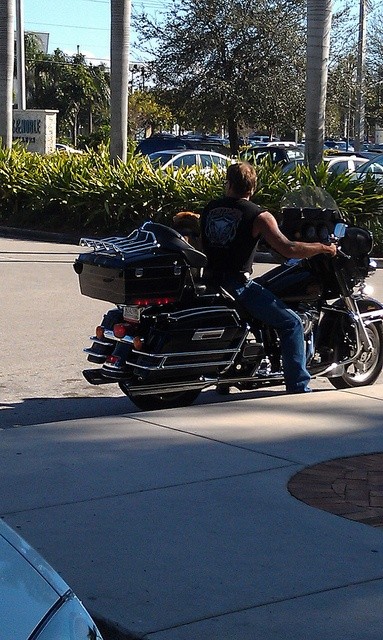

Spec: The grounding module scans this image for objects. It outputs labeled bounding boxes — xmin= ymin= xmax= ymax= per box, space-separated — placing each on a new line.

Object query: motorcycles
xmin=73 ymin=185 xmax=382 ymax=410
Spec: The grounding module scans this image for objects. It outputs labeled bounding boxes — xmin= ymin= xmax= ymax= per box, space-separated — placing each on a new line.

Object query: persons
xmin=199 ymin=162 xmax=336 ymax=393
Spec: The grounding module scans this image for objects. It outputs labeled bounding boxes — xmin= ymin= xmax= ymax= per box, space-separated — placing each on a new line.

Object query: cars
xmin=137 ymin=149 xmax=236 ymax=181
xmin=134 ymin=140 xmax=187 ymax=152
xmin=282 ymin=157 xmax=381 ymax=186
xmin=240 ymin=145 xmax=303 ymax=166
xmin=55 ymin=143 xmax=82 ymax=155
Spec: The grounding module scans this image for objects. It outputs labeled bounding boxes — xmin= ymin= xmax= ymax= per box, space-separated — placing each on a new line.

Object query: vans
xmin=248 ymin=136 xmax=277 ymax=142
xmin=325 ymin=141 xmax=355 ymax=151
